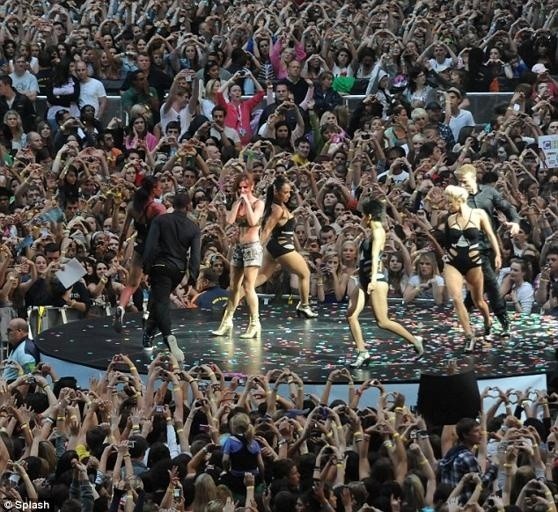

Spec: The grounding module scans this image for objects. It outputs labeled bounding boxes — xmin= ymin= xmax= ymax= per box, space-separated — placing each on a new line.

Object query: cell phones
xmin=174 ymin=488 xmax=180 ymax=501
xmin=27 ymin=377 xmax=36 ymax=384
xmin=155 ymin=406 xmax=165 ymax=413
xmin=41 ymin=227 xmax=48 ymax=238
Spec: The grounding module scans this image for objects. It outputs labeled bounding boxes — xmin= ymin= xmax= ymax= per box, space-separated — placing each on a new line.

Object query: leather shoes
xmin=499 ymin=313 xmax=510 ymax=331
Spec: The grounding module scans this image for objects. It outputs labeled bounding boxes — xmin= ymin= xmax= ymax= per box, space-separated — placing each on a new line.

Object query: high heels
xmin=113 ymin=306 xmax=125 ymax=331
xmin=351 ymin=351 xmax=371 ymax=369
xmin=412 ymin=336 xmax=427 ymax=361
xmin=142 ymin=311 xmax=149 ymax=327
xmin=296 ymin=301 xmax=319 ymax=318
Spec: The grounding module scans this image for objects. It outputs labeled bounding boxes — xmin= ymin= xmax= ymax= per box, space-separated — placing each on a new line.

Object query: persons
xmin=0 ymin=1 xmax=558 ymax=512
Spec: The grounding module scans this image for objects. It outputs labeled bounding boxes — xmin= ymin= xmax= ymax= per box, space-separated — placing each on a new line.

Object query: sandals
xmin=483 ymin=320 xmax=493 ymax=341
xmin=463 ymin=335 xmax=477 ymax=351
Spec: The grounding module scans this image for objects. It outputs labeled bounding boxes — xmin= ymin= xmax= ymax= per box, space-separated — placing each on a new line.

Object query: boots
xmin=210 ymin=307 xmax=233 ymax=336
xmin=142 ymin=331 xmax=154 ymax=350
xmin=238 ymin=313 xmax=262 ymax=339
xmin=162 ymin=334 xmax=185 ymax=362
